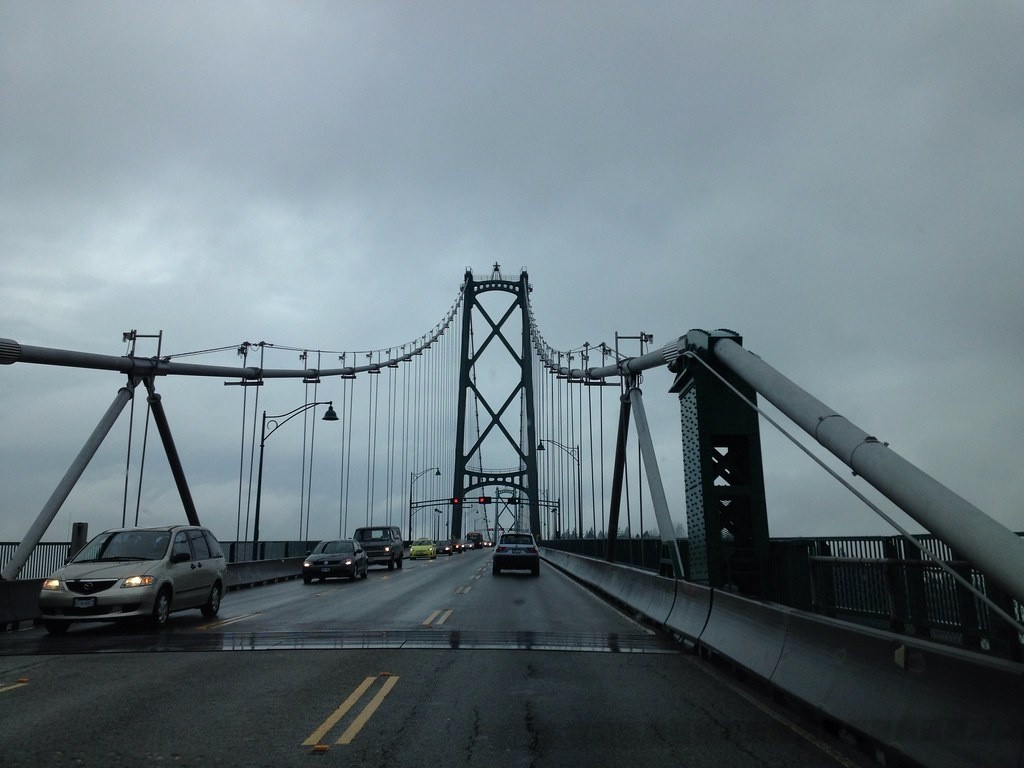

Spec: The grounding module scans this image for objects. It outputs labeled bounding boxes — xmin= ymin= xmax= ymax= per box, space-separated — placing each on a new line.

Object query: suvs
xmin=492 ymin=533 xmax=540 ymax=577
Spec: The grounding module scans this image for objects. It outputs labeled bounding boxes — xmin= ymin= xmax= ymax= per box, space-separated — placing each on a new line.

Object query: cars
xmin=302 ymin=540 xmax=368 ymax=583
xmin=37 ymin=526 xmax=228 ymax=638
xmin=462 ymin=540 xmax=474 ymax=550
xmin=434 ymin=540 xmax=453 ymax=557
xmin=409 ymin=538 xmax=436 ymax=559
xmin=449 ymin=538 xmax=463 ymax=553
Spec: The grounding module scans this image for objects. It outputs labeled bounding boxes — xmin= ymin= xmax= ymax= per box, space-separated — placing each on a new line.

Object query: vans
xmin=349 ymin=525 xmax=406 ymax=571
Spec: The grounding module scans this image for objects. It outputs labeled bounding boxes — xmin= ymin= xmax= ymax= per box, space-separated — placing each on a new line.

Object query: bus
xmin=464 ymin=532 xmax=484 ymax=550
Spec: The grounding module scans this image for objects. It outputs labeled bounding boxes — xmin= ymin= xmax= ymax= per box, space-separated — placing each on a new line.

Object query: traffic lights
xmin=478 ymin=497 xmax=491 ymax=504
xmin=450 ymin=498 xmax=463 ymax=504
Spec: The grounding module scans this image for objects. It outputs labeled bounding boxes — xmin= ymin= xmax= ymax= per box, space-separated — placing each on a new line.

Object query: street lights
xmin=536 ymin=439 xmax=582 ymax=538
xmin=252 ymin=401 xmax=339 ymax=560
xmin=408 ymin=467 xmax=441 ymax=545
